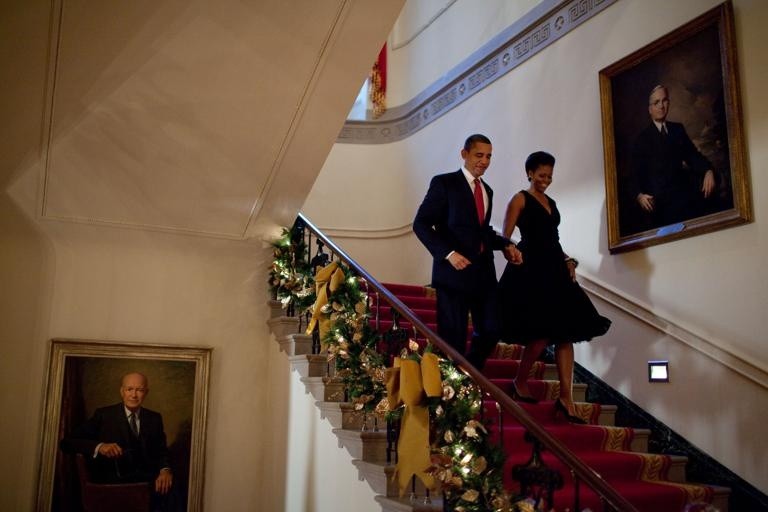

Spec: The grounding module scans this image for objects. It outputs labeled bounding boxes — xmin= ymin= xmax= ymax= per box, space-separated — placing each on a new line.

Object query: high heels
xmin=509 ymin=379 xmax=537 ymax=403
xmin=554 ymin=397 xmax=588 ymax=425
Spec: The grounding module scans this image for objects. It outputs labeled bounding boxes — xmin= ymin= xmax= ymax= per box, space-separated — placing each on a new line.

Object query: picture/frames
xmin=596 ymin=1 xmax=754 ymax=258
xmin=31 ymin=336 xmax=215 ymax=510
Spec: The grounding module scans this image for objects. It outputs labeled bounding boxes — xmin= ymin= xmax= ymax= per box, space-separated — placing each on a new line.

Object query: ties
xmin=661 ymin=124 xmax=666 ymax=137
xmin=129 ymin=413 xmax=138 ymax=436
xmin=473 ymin=179 xmax=485 ymax=254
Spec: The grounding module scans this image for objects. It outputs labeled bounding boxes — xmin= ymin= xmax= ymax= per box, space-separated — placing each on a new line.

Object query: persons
xmin=68 ymin=372 xmax=181 ymax=511
xmin=499 ymin=149 xmax=612 ymax=425
xmin=630 ymin=84 xmax=722 ymax=232
xmin=413 ymin=133 xmax=522 ymax=373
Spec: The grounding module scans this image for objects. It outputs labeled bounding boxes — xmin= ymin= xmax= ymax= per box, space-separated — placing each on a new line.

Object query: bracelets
xmin=565 ymin=258 xmax=578 ymax=267
